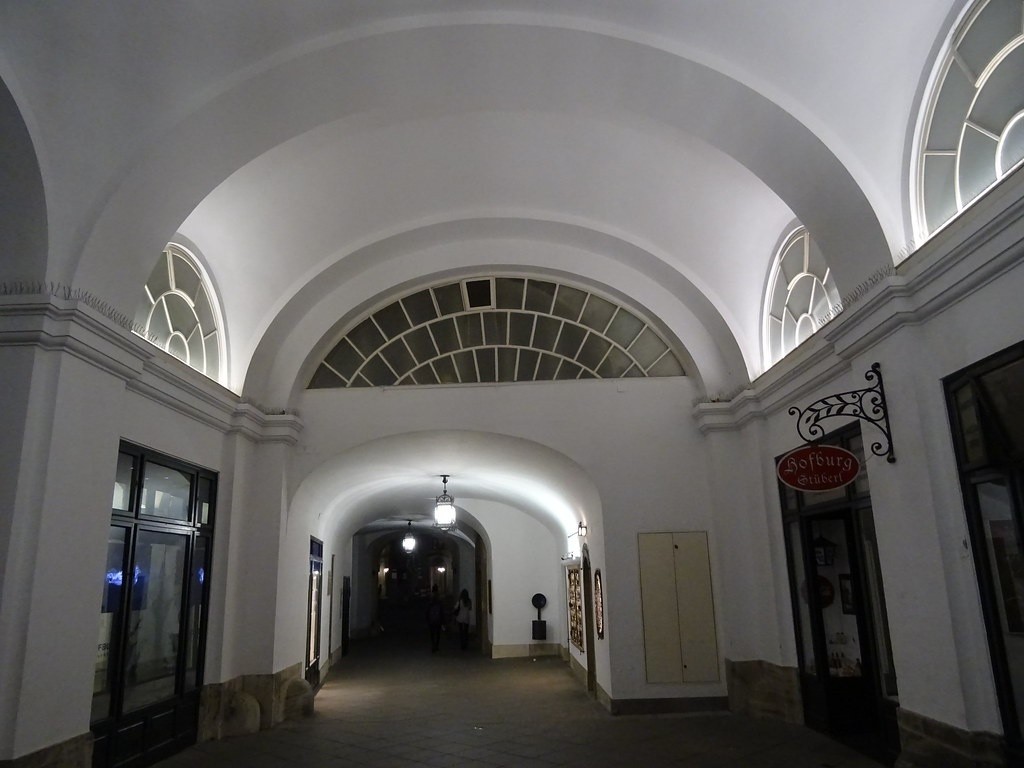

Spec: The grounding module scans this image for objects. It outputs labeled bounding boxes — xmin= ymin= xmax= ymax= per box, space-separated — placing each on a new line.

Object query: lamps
xmin=401 ymin=520 xmax=416 ymax=554
xmin=578 ymin=522 xmax=587 ymax=537
xmin=433 ymin=474 xmax=458 ymax=534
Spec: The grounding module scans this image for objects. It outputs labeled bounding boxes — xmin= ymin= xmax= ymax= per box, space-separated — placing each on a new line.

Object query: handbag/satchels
xmin=441 ymin=621 xmax=447 ymax=632
xmin=455 ymin=600 xmax=460 ymax=616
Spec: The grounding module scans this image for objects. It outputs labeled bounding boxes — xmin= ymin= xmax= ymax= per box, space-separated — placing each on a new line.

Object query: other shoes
xmin=432 ymin=647 xmax=440 ymax=654
xmin=461 ymin=642 xmax=468 ymax=648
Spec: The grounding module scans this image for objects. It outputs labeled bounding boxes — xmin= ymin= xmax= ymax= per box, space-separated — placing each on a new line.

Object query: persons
xmin=425 ymin=592 xmax=446 ymax=652
xmin=452 ymin=589 xmax=472 ymax=650
